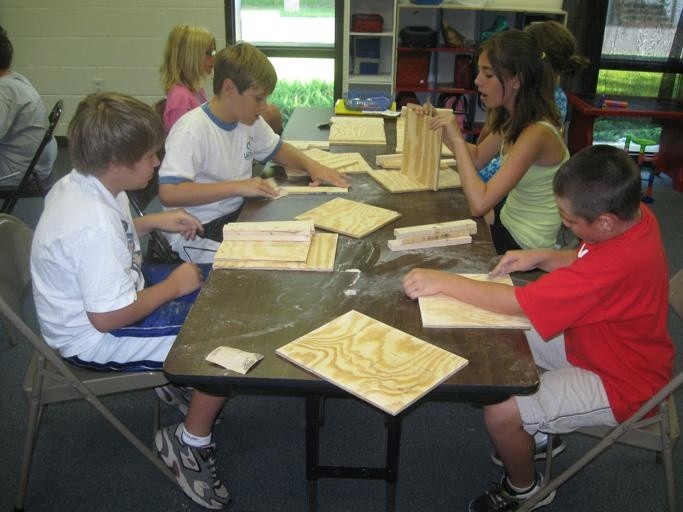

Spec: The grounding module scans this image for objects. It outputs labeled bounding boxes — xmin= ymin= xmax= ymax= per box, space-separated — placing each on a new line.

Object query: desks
xmin=160 ymin=103 xmax=542 ymax=511
xmin=561 ymin=86 xmax=682 ymax=194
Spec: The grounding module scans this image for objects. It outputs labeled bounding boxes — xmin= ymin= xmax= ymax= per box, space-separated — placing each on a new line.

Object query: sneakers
xmin=468 ymin=473 xmax=556 ymax=512
xmin=155 ymin=383 xmax=224 ymax=425
xmin=490 ymin=434 xmax=567 ymax=467
xmin=154 ymin=425 xmax=232 ymax=510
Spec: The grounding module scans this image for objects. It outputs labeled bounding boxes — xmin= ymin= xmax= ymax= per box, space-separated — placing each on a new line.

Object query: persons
xmin=157 ymin=24 xmax=283 ymax=138
xmin=24 ymin=91 xmax=233 ymax=509
xmin=411 ymin=26 xmax=570 ymax=256
xmin=477 ymin=21 xmax=592 ymax=188
xmin=156 ymin=42 xmax=352 ymax=265
xmin=1 ymin=26 xmax=60 ymax=186
xmin=399 ymin=144 xmax=676 ymax=511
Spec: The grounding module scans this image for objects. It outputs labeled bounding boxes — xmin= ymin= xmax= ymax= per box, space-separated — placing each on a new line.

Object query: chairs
xmin=123 ymin=145 xmax=190 ymax=264
xmin=507 ymin=259 xmax=682 ymax=512
xmin=2 ymin=96 xmax=68 ymax=220
xmin=1 ymin=209 xmax=227 ymax=511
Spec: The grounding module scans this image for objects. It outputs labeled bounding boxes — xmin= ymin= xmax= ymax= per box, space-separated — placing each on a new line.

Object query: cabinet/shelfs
xmin=395 ymin=0 xmax=572 ymax=140
xmin=341 ymin=1 xmax=394 ymax=101
